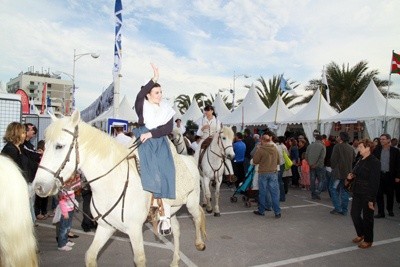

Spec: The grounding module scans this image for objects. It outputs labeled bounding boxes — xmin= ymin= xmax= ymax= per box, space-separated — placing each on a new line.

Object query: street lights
xmin=231 ymin=70 xmax=249 ymax=111
xmin=72 ymin=49 xmax=102 ymax=115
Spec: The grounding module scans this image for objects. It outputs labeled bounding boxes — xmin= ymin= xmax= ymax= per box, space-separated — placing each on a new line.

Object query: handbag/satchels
xmin=283 ymin=150 xmax=293 ymax=171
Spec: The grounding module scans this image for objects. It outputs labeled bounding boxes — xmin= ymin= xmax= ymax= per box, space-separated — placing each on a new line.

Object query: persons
xmin=19 ymin=123 xmax=58 ymax=220
xmin=132 ymin=62 xmax=175 ymax=234
xmin=1 ymin=122 xmax=32 ymax=185
xmin=232 ymin=125 xmax=400 ymax=248
xmin=81 ymin=190 xmax=98 ymax=233
xmin=53 ymin=179 xmax=80 ymax=251
xmin=194 ymin=105 xmax=237 ymax=182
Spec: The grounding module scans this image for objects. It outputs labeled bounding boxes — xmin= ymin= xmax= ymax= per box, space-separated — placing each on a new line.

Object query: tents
xmin=158 ymin=78 xmax=400 ymax=144
xmin=89 ymin=96 xmax=139 ymax=131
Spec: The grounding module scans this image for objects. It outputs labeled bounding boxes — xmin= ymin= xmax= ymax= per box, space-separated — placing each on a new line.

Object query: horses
xmin=193 ymin=121 xmax=236 ymax=217
xmin=1 ymin=154 xmax=40 ymax=266
xmin=33 ymin=109 xmax=207 ymax=266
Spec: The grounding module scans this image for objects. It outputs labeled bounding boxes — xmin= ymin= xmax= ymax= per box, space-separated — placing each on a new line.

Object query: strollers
xmin=231 ymin=160 xmax=261 ymax=208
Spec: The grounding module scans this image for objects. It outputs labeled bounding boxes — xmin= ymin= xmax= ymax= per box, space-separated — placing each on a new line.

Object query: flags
xmin=113 ymin=0 xmax=123 ymax=82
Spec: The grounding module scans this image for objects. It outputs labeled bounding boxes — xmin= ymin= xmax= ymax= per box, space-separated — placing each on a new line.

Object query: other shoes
xmin=161 ymin=218 xmax=172 ymax=234
xmin=68 ymin=234 xmax=79 ymax=238
xmin=352 ymin=236 xmax=364 ymax=243
xmin=57 ymin=245 xmax=72 ymax=252
xmin=330 ymin=209 xmax=347 ymax=216
xmin=358 ymin=241 xmax=372 ymax=248
xmin=374 ymin=215 xmax=385 ymax=218
xmin=66 ymin=241 xmax=75 ymax=246
xmin=253 ymin=211 xmax=265 ymax=216
xmin=388 ymin=212 xmax=394 ymax=217
xmin=311 ymin=190 xmax=321 ymax=200
xmin=275 ymin=213 xmax=281 ymax=218
xmin=56 ymin=237 xmax=72 ymax=242
